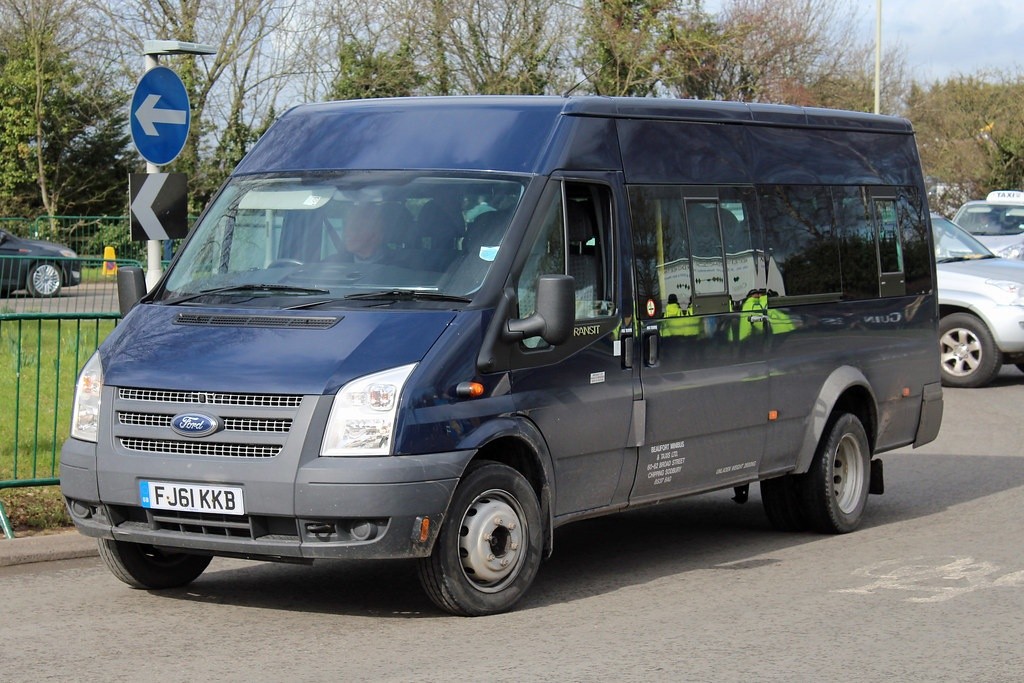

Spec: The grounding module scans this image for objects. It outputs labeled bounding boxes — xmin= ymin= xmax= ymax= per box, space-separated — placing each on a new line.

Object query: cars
xmin=0 ymin=227 xmax=82 ymax=298
xmin=703 ymin=190 xmax=1024 ymax=388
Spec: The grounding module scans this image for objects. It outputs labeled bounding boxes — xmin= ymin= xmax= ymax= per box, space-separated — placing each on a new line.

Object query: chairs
xmin=445 ymin=211 xmax=549 ymax=315
xmin=546 ymin=198 xmax=602 ymax=317
xmin=383 ymin=203 xmax=424 ymax=269
xmin=1000 ymin=216 xmax=1021 ymax=232
xmin=417 ymin=198 xmax=467 ymax=272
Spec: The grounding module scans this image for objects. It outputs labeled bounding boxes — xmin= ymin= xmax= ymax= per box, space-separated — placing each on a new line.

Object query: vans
xmin=59 ymin=94 xmax=944 ymax=618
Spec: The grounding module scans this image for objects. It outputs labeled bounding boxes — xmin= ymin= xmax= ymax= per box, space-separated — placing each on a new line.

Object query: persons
xmin=321 ymin=204 xmax=385 ymax=264
xmin=608 ymin=306 xmax=640 ymax=341
xmin=659 ymin=293 xmax=796 ymax=341
